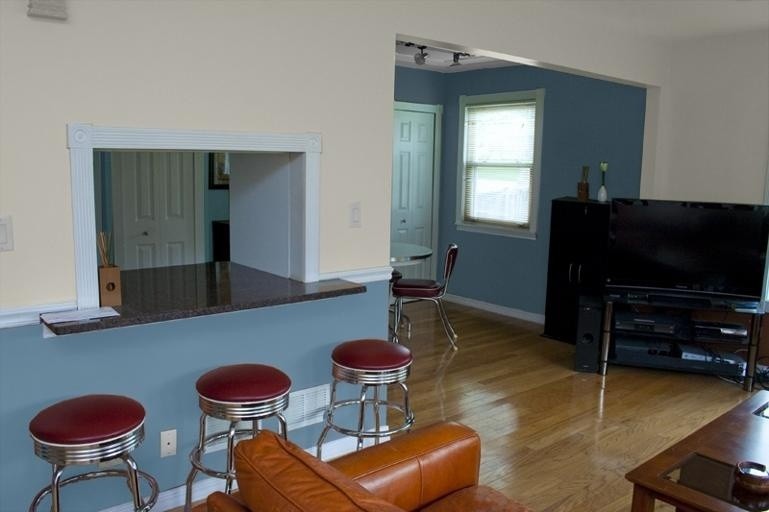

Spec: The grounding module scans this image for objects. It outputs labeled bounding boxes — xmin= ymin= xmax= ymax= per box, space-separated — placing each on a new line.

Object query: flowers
xmin=601 ymin=161 xmax=609 ymax=185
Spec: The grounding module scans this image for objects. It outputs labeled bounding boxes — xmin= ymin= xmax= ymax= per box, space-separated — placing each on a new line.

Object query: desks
xmin=390 ymin=244 xmax=434 ymax=333
xmin=625 ymin=387 xmax=769 ymax=512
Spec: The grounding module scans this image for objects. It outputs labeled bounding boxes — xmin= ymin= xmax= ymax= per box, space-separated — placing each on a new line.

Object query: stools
xmin=186 ymin=365 xmax=293 ymax=512
xmin=316 ymin=337 xmax=412 ymax=454
xmin=28 ymin=395 xmax=160 ymax=512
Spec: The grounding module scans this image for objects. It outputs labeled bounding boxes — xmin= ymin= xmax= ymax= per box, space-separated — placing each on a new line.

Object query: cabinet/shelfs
xmin=598 ymin=293 xmax=765 ymax=392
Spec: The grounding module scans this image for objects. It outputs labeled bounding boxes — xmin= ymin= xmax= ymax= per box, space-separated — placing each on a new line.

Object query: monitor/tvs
xmin=605 ymin=197 xmax=769 ymax=308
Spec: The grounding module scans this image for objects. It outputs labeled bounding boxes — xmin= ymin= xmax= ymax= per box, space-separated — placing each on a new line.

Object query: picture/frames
xmin=207 ymin=152 xmax=229 ymax=190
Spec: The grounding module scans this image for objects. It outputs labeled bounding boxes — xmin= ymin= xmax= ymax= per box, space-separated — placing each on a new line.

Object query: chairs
xmin=197 ymin=423 xmax=530 ymax=512
xmin=391 ymin=244 xmax=459 ymax=352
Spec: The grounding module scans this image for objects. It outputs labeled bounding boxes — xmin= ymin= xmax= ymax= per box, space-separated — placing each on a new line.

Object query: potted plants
xmin=577 ymin=166 xmax=590 ymax=200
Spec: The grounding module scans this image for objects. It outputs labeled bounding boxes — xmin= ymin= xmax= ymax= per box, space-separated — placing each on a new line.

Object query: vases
xmin=598 ymin=187 xmax=609 ymax=202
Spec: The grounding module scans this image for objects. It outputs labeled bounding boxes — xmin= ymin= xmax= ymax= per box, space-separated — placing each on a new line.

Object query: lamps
xmin=413 ymin=46 xmax=428 ymax=66
xmin=448 ymin=54 xmax=463 ymax=69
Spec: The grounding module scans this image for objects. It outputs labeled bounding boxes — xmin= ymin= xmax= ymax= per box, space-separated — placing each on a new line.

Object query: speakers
xmin=574 ymin=295 xmax=602 ymax=372
